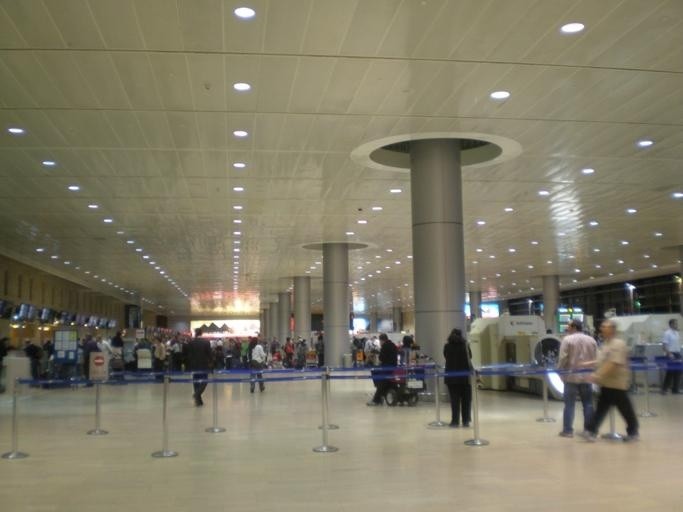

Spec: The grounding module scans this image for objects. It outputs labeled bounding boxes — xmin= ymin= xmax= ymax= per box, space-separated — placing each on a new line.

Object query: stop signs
xmin=92 ymin=356 xmax=104 ymax=366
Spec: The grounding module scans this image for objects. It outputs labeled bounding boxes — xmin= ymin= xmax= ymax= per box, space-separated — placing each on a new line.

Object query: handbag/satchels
xmin=250 ymin=360 xmax=264 ymax=370
xmin=469 ymin=367 xmax=480 ymax=385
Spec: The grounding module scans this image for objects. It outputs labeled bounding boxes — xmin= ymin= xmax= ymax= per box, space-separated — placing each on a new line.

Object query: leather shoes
xmin=192 ymin=393 xmax=203 ymax=406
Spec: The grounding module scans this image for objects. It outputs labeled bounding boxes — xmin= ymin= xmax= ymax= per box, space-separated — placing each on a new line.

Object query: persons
xmin=444 ymin=327 xmax=473 ymax=427
xmin=250 ymin=337 xmax=265 ymax=393
xmin=184 ymin=328 xmax=213 ymax=406
xmin=0 ymin=329 xmax=323 ymax=374
xmin=663 ymin=318 xmax=683 ymax=395
xmin=351 ymin=333 xmax=381 ymax=367
xmin=366 ymin=334 xmax=413 ymax=405
xmin=555 ymin=318 xmax=638 ymax=442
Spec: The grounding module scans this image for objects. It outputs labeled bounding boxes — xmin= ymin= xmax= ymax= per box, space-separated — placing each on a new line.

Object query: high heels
xmin=249 ymin=386 xmax=265 ymax=393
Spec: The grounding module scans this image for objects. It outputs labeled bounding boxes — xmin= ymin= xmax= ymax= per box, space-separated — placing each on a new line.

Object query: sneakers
xmin=559 ymin=431 xmax=640 ymax=442
xmin=367 ymin=400 xmax=379 ymax=406
xmin=659 ymin=390 xmax=683 ymax=395
xmin=448 ymin=423 xmax=468 ymax=428
